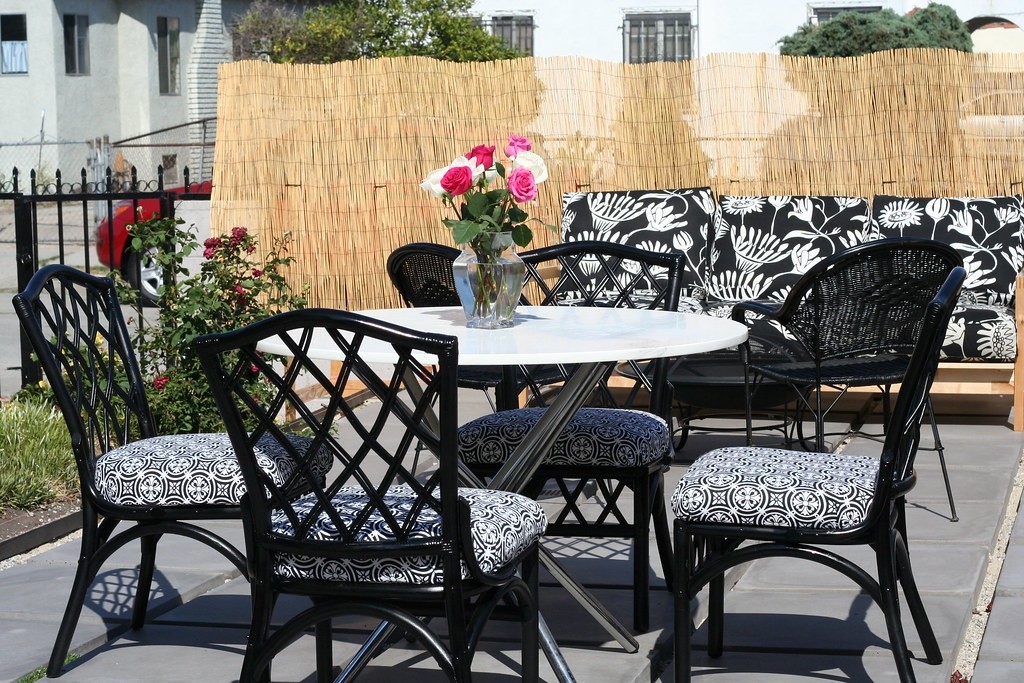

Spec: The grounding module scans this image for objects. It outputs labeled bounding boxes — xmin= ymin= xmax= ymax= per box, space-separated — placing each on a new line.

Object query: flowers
xmin=419 ymin=133 xmax=557 ymax=318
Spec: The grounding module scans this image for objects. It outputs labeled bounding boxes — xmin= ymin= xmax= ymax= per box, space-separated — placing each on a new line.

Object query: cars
xmin=94 ymin=177 xmax=212 ymax=307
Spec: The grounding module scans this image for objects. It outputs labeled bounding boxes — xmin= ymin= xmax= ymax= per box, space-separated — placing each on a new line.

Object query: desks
xmin=255 ymin=306 xmax=749 ymax=683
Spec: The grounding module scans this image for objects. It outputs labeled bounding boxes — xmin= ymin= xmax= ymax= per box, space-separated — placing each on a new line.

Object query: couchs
xmin=519 ymin=186 xmax=1024 ymax=431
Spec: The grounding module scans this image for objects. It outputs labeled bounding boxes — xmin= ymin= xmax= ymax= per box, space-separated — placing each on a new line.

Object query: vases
xmin=452 ymin=231 xmax=525 ymax=330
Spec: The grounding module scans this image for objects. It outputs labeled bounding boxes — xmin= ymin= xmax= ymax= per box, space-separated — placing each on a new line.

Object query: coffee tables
xmin=613 ymin=355 xmax=817 ymax=453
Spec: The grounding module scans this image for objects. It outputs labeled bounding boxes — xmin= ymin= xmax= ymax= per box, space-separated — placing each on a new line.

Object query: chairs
xmin=193 ymin=308 xmax=547 ymax=683
xmin=386 ymin=242 xmax=574 ymax=478
xmin=11 ymin=264 xmax=333 ymax=683
xmin=457 ymin=241 xmax=687 ymax=634
xmin=670 ymin=267 xmax=967 ymax=683
xmin=732 ymin=237 xmax=964 ymax=522
